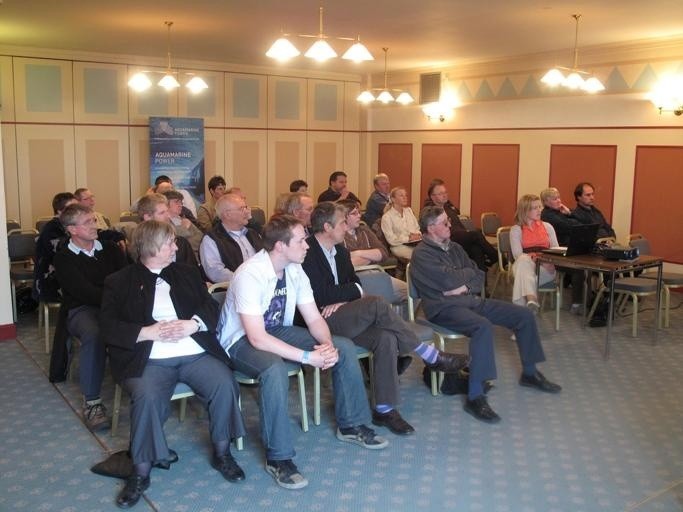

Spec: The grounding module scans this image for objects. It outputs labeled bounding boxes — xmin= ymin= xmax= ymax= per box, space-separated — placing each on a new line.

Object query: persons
xmin=408 ymin=203 xmax=564 ymax=424
xmin=379 ymin=188 xmax=422 ymax=261
xmin=34 ymin=187 xmax=127 ymax=305
xmin=292 ymin=199 xmax=473 ymax=437
xmin=47 ymin=201 xmax=131 ymax=432
xmin=285 ymin=172 xmax=390 ymax=267
xmin=141 ymin=176 xmax=207 ymax=291
xmin=538 ymin=186 xmax=594 ymax=317
xmin=200 ymin=175 xmax=267 ymax=287
xmin=97 ymin=216 xmax=248 ymax=508
xmin=569 ymin=181 xmax=645 ymax=304
xmin=214 ymin=211 xmax=391 ymax=493
xmin=507 ymin=193 xmax=560 ymax=343
xmin=418 ymin=178 xmax=501 ymax=271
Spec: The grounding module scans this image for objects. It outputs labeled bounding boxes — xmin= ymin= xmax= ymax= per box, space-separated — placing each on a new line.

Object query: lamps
xmin=355 ymin=44 xmax=415 ymax=107
xmin=258 ymin=2 xmax=378 ymax=67
xmin=122 ymin=20 xmax=212 ymax=97
xmin=540 ymin=12 xmax=607 ymax=97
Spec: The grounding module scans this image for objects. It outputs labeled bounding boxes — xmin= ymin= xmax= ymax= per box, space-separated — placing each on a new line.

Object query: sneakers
xmin=568 ymin=303 xmax=590 ymax=316
xmin=264 ymin=458 xmax=309 ymax=490
xmin=336 ymin=422 xmax=390 ymax=451
xmin=79 ymin=396 xmax=113 ymax=433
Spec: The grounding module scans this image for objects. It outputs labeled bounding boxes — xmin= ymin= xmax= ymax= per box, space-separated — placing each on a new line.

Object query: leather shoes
xmin=461 ymin=394 xmax=502 ymax=424
xmin=114 ymin=473 xmax=151 ymax=510
xmin=518 ymin=368 xmax=564 ymax=395
xmin=209 ymin=451 xmax=246 ymax=483
xmin=372 ymin=406 xmax=417 ymax=436
xmin=526 ymin=299 xmax=541 ymax=315
xmin=422 ymin=349 xmax=473 ymax=374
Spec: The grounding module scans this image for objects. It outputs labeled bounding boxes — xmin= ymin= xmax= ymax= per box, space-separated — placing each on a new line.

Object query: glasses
xmin=426 ymin=216 xmax=451 ymax=227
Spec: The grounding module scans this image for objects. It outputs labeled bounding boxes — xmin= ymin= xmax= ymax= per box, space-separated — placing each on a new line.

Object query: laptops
xmin=541 ymin=222 xmax=601 ymax=256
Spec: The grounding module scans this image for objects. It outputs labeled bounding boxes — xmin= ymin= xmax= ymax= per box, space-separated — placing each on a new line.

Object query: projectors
xmin=600 ymin=247 xmax=640 ymax=260
